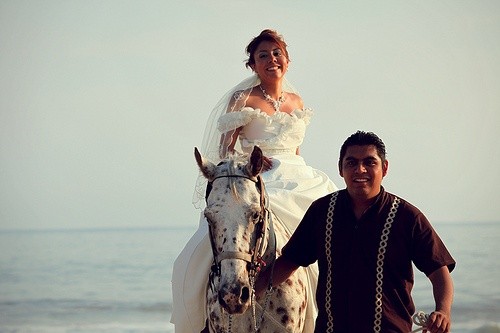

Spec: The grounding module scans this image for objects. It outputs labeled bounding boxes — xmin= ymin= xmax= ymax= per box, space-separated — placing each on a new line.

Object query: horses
xmin=193 ymin=144 xmax=320 ymax=333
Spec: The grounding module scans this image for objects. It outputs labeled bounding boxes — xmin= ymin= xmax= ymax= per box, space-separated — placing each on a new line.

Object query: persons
xmin=167 ymin=27 xmax=337 ymax=333
xmin=250 ymin=128 xmax=458 ymax=333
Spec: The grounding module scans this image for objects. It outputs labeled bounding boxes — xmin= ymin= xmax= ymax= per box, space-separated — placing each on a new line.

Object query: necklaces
xmin=259 ymin=84 xmax=286 ymax=112
